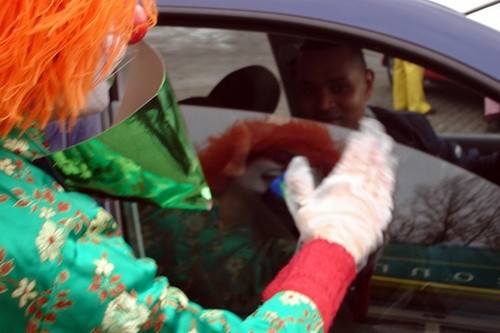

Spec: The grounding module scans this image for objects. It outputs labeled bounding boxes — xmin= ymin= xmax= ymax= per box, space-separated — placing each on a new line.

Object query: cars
xmin=98 ymin=1 xmax=500 ymax=331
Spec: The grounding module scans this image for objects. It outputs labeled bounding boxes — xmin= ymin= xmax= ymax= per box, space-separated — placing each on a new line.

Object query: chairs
xmin=176 ymin=66 xmax=281 ymax=114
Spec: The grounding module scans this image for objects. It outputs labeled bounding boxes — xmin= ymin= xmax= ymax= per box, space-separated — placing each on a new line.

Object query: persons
xmin=391 ymin=54 xmax=436 ymax=116
xmin=296 ymin=35 xmax=464 ymax=182
xmin=171 ymin=120 xmax=341 ymax=307
xmin=0 ymin=0 xmax=395 ymax=333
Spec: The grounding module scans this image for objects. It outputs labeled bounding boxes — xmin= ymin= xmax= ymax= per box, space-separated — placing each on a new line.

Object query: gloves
xmin=281 ymin=117 xmax=397 ymax=272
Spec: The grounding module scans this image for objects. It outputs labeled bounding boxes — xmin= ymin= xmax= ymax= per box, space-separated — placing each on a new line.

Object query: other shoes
xmin=427 ymin=106 xmax=438 ymax=115
